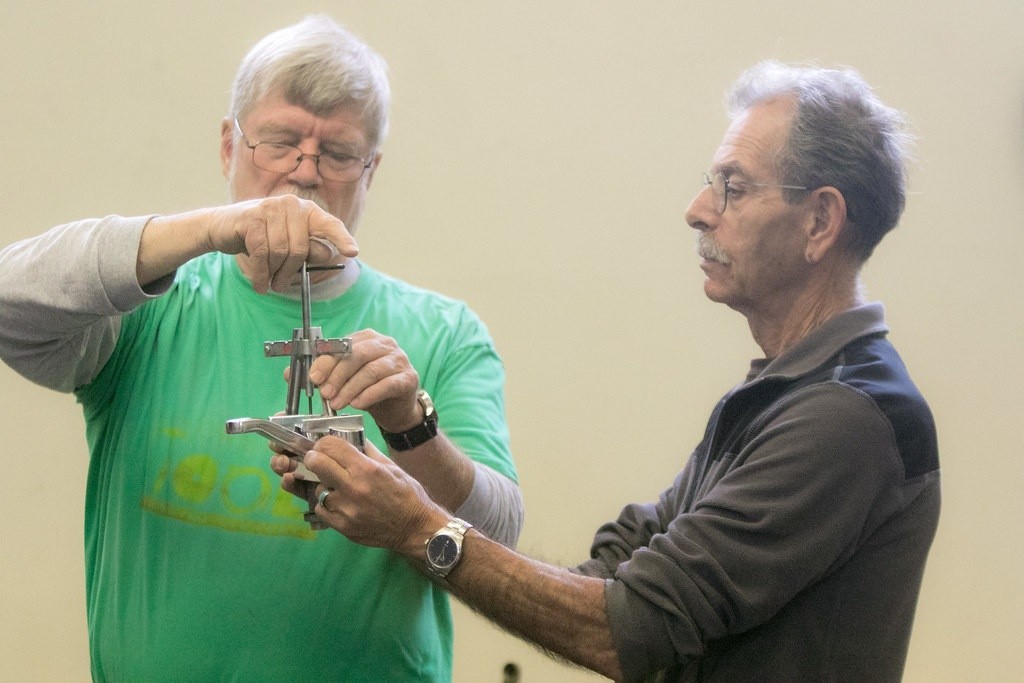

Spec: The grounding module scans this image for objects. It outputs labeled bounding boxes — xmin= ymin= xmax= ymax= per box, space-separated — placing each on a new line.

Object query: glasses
xmin=701 ymin=171 xmax=857 ymax=224
xmin=234 ymin=119 xmax=377 ymax=184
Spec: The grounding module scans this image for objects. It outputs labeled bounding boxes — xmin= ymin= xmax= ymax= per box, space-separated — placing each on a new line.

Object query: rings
xmin=318 ymin=490 xmax=331 ymax=506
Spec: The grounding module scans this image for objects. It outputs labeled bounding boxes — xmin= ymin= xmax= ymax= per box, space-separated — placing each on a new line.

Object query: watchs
xmin=425 ymin=517 xmax=474 ymax=583
xmin=376 ymin=386 xmax=438 ymax=452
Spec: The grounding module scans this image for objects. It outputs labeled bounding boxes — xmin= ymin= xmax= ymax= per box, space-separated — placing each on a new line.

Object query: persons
xmin=0 ymin=14 xmax=525 ymax=683
xmin=269 ymin=66 xmax=942 ymax=683
